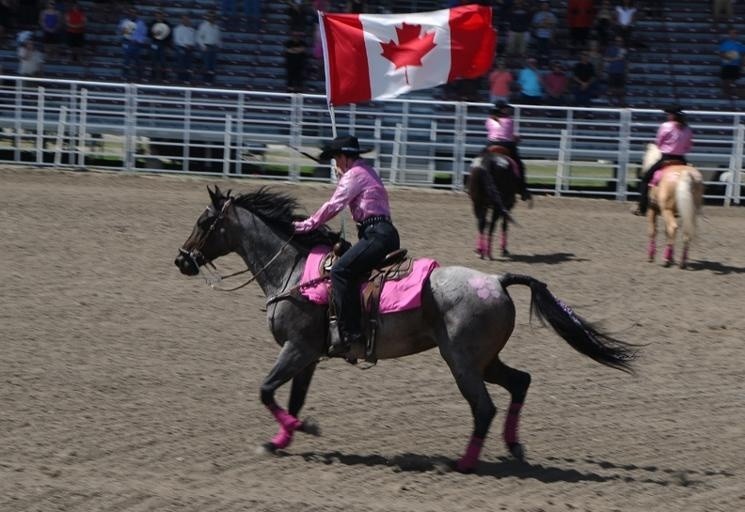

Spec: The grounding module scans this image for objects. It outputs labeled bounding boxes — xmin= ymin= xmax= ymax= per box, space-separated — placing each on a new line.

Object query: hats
xmin=663 ymin=103 xmax=686 ymax=118
xmin=151 ymin=22 xmax=170 ymax=40
xmin=487 ymin=99 xmax=515 ymax=117
xmin=318 ymin=133 xmax=374 ymax=159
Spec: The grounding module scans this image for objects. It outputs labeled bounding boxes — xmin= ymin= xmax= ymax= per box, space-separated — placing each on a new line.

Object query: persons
xmin=488 ymin=0 xmax=638 ymax=104
xmin=631 ymin=104 xmax=690 ymax=218
xmin=285 ymin=0 xmax=316 ymax=92
xmin=485 ymin=100 xmax=531 ymax=200
xmin=291 ymin=135 xmax=400 ymax=359
xmin=718 ymin=27 xmax=745 ymax=98
xmin=118 ymin=9 xmax=220 ymax=83
xmin=16 ymin=1 xmax=88 ymax=87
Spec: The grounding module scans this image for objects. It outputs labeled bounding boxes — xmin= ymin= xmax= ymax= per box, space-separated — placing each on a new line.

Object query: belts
xmin=360 ymin=214 xmax=387 ymax=225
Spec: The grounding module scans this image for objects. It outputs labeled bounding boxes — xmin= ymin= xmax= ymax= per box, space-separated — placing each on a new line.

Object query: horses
xmin=642 ymin=143 xmax=704 ymax=270
xmin=174 ymin=185 xmax=653 ymax=473
xmin=468 ymin=135 xmax=525 ymax=260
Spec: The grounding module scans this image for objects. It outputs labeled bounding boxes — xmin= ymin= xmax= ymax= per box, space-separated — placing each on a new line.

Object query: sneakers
xmin=338 ymin=335 xmax=367 ymax=361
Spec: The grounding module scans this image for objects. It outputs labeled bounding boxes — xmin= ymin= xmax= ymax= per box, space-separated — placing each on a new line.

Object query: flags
xmin=318 ymin=4 xmax=494 ymax=105
